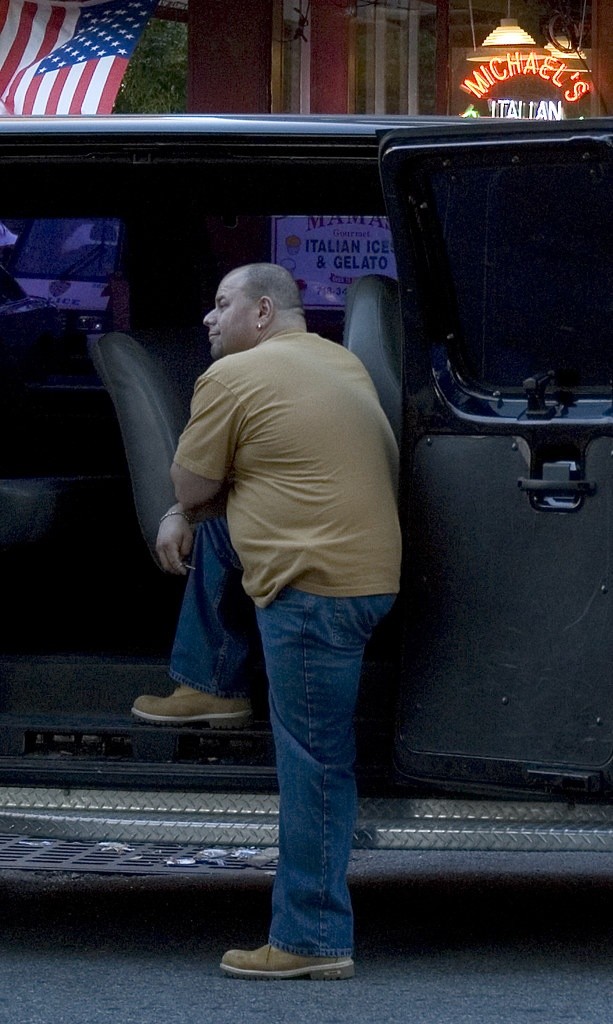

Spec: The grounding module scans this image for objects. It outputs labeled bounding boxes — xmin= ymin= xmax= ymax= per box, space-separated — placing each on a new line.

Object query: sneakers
xmin=129 ymin=681 xmax=254 ymax=728
xmin=219 ymin=943 xmax=355 ymax=981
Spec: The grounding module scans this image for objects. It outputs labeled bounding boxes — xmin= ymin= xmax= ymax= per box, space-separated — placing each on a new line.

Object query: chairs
xmin=100 ymin=332 xmax=190 ymax=562
xmin=342 ymin=272 xmax=404 ymax=446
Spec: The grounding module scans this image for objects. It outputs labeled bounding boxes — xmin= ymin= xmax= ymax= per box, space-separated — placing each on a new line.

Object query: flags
xmin=0 ymin=0 xmax=154 ymax=116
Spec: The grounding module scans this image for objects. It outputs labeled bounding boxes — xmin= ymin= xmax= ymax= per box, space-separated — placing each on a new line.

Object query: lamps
xmin=544 ymin=32 xmax=587 ymax=60
xmin=481 ymin=0 xmax=537 ymax=46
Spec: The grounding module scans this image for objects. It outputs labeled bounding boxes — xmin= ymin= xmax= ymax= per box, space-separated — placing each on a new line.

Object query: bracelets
xmin=159 ymin=511 xmax=190 ymax=523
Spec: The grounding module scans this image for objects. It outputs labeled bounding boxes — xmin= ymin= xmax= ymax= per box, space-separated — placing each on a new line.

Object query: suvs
xmin=1 ymin=113 xmax=613 ymax=892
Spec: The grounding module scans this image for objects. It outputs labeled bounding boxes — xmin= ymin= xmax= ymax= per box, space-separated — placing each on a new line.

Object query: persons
xmin=133 ymin=262 xmax=402 ymax=981
